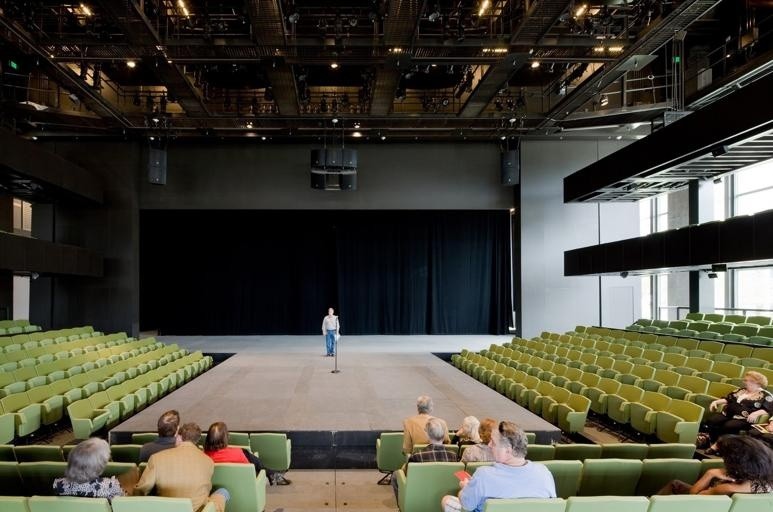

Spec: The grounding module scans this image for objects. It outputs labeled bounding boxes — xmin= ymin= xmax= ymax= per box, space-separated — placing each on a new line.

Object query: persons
xmin=200 ymin=423 xmax=289 ymax=487
xmin=322 ymin=308 xmax=340 ymax=356
xmin=403 ymin=395 xmax=451 ymax=454
xmin=461 ymin=419 xmax=500 ymax=464
xmin=132 ymin=424 xmax=230 ymax=512
xmin=390 ymin=418 xmax=459 ymax=512
xmin=134 ymin=410 xmax=182 ymax=466
xmin=653 ymin=435 xmax=773 ymax=497
xmin=706 ymin=413 xmax=772 ymax=458
xmin=452 ymin=415 xmax=481 ymax=457
xmin=698 ymin=371 xmax=772 ymax=445
xmin=442 ymin=423 xmax=557 ymax=512
xmin=53 ymin=437 xmax=126 ymax=499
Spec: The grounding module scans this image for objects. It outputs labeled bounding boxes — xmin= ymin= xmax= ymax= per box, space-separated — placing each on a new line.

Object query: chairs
xmin=250 ymin=433 xmax=291 ymax=486
xmin=729 ymin=493 xmax=773 ymax=512
xmin=466 ymin=461 xmax=496 ymax=477
xmin=524 ymin=444 xmax=555 ymax=461
xmin=0 ymin=462 xmax=27 ymax=496
xmin=648 ymin=443 xmax=696 ymax=458
xmin=566 ymin=495 xmax=650 ymax=512
xmin=554 ymin=444 xmax=602 ymax=463
xmin=227 ymin=432 xmax=251 ymax=452
xmin=210 ymin=463 xmax=266 ymax=512
xmin=28 ymin=496 xmax=112 ymax=512
xmin=18 ymin=461 xmax=69 ymax=495
xmin=110 ymin=444 xmax=143 ymax=466
xmin=698 ymin=459 xmax=726 ymax=480
xmin=602 ymin=443 xmax=648 ymax=461
xmin=0 ymin=445 xmax=16 ymax=461
xmin=101 ymin=462 xmax=136 ymax=479
xmin=647 ymin=495 xmax=733 ymax=512
xmin=484 ymin=498 xmax=566 ymax=512
xmin=577 ymin=458 xmax=643 ymax=496
xmin=376 ymin=433 xmax=406 ymax=485
xmin=63 ymin=445 xmax=77 ymax=462
xmin=402 ymin=444 xmax=459 ymax=462
xmin=139 ymin=462 xmax=147 ymax=479
xmin=14 ymin=444 xmax=65 ymax=462
xmin=111 ymin=496 xmax=193 ymax=512
xmin=0 ymin=496 xmax=31 ymax=512
xmin=459 ymin=445 xmax=474 ymax=459
xmin=397 ymin=462 xmax=465 ymax=512
xmin=532 ymin=460 xmax=583 ymax=500
xmin=0 ymin=320 xmax=213 ymax=445
xmin=634 ymin=458 xmax=703 ymax=498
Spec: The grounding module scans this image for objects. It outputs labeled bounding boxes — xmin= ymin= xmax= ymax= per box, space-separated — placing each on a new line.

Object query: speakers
xmin=501 ymin=149 xmax=519 ymax=187
xmin=145 ymin=148 xmax=168 ymax=184
xmin=339 ymin=174 xmax=357 ymax=190
xmin=343 ymin=149 xmax=358 ymax=168
xmin=326 ymin=148 xmax=343 ymax=167
xmin=311 ymin=149 xmax=325 ymax=169
xmin=311 ymin=174 xmax=329 ymax=190
